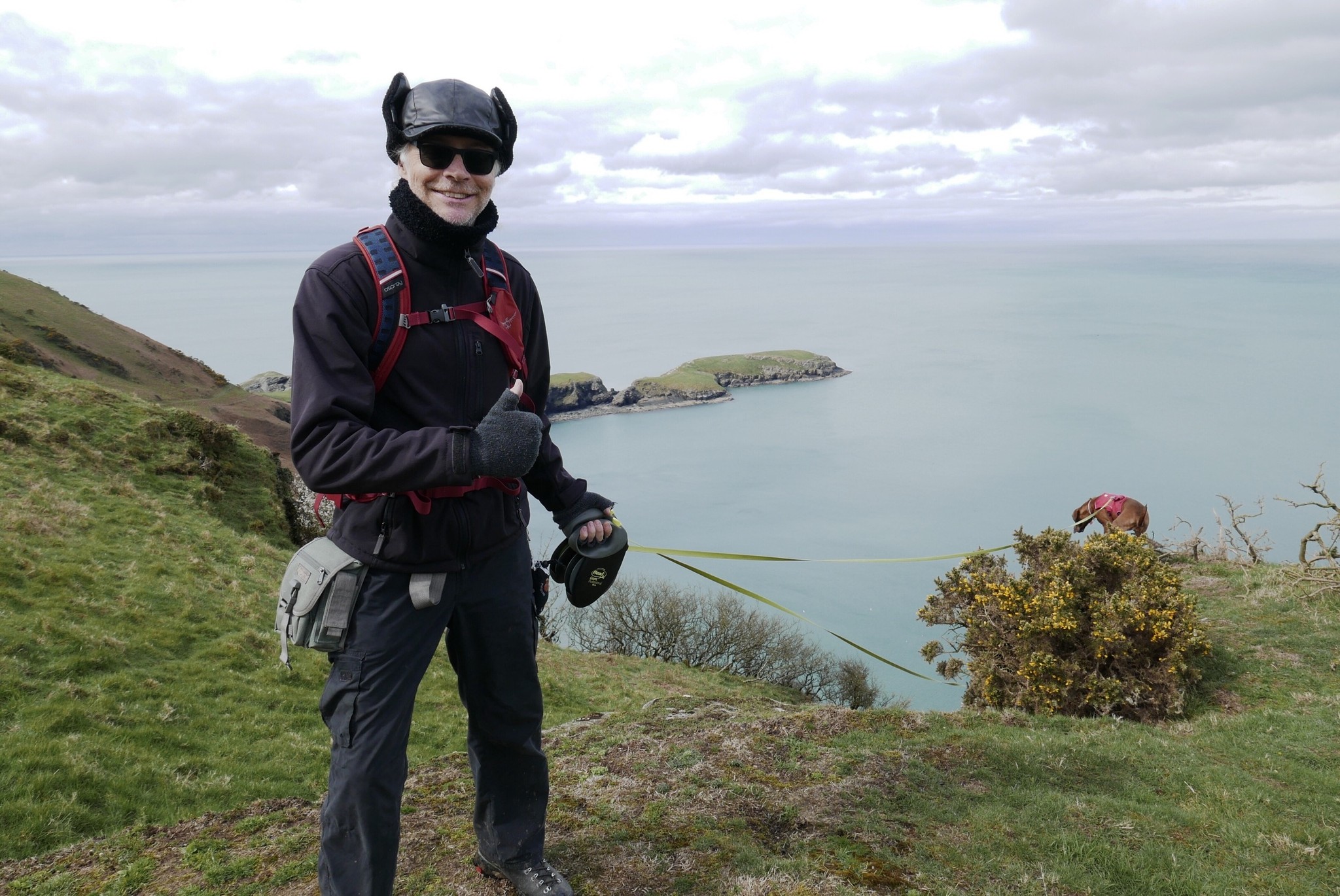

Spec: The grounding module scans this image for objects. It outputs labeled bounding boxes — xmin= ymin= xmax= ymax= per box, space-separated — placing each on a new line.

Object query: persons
xmin=289 ymin=72 xmax=616 ymax=896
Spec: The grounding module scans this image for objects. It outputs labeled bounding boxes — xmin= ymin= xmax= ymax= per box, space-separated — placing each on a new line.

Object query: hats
xmin=381 ymin=72 xmax=518 ymax=177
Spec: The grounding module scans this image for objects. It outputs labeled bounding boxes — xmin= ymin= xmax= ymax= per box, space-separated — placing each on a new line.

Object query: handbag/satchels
xmin=273 ymin=536 xmax=371 ymax=670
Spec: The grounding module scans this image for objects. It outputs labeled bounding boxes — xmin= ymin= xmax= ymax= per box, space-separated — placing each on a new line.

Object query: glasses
xmin=408 ymin=139 xmax=501 ymax=176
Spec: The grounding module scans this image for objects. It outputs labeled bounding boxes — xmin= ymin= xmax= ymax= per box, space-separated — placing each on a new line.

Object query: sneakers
xmin=471 ymin=848 xmax=572 ymax=896
